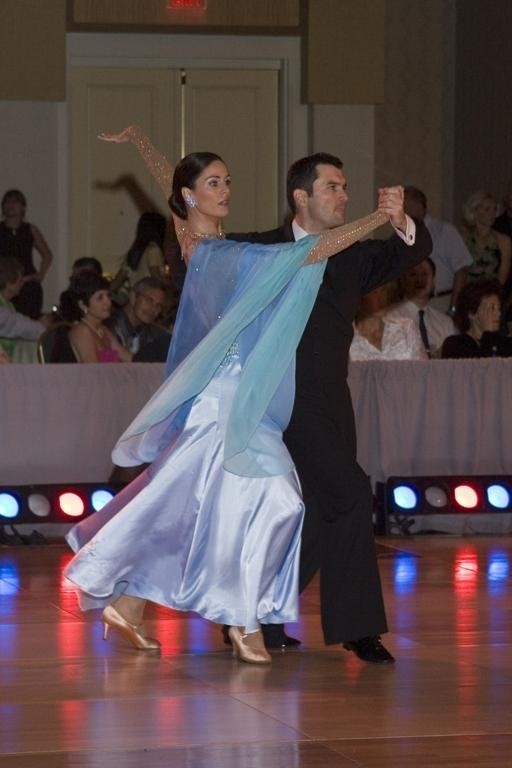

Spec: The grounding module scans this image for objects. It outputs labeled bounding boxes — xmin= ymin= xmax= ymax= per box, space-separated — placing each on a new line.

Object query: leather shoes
xmin=341 ymin=633 xmax=397 ymax=666
xmin=261 ymin=623 xmax=303 ymax=649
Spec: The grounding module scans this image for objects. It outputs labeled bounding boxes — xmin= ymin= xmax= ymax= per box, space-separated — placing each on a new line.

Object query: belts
xmin=426 ymin=289 xmax=454 ymax=298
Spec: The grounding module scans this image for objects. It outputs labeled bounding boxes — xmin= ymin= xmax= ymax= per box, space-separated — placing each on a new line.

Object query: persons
xmin=1 ymin=189 xmax=187 ymax=363
xmin=349 ymin=183 xmax=511 ymax=359
xmin=63 ymin=123 xmax=402 ymax=667
xmin=220 ymin=148 xmax=435 ymax=665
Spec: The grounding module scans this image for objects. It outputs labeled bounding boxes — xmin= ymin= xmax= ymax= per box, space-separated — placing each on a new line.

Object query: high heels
xmin=99 ymin=604 xmax=163 ymax=652
xmin=226 ymin=623 xmax=273 ymax=668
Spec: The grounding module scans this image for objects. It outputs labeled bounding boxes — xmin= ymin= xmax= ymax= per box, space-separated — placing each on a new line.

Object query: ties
xmin=417 ymin=308 xmax=432 ymax=360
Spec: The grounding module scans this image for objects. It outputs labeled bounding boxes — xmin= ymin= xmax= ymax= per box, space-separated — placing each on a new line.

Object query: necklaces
xmin=180 ymin=225 xmax=226 ymax=242
